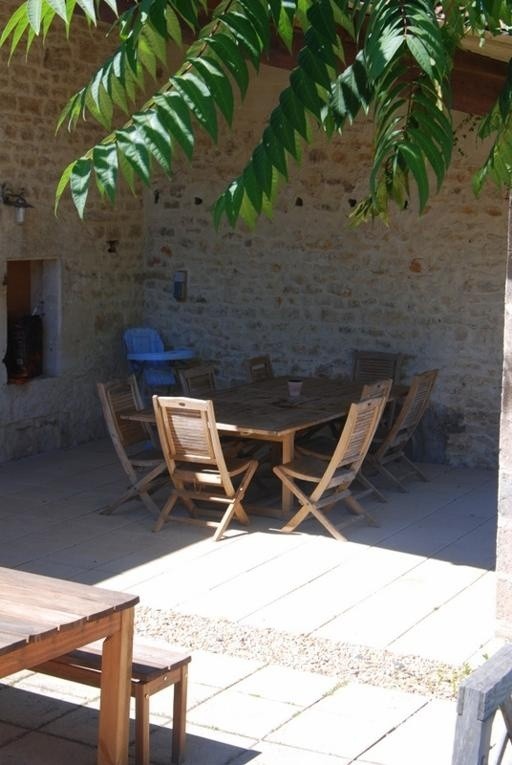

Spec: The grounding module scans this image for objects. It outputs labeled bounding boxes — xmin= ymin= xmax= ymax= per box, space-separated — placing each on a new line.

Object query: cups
xmin=287 ymin=378 xmax=303 ymax=396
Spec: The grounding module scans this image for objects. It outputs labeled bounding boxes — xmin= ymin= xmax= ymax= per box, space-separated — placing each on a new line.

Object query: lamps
xmin=1 ymin=180 xmax=35 ymax=225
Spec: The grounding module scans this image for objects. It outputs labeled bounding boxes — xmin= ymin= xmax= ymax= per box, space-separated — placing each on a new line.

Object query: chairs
xmin=122 ymin=327 xmax=193 ymax=396
xmin=151 ymin=394 xmax=259 ymax=541
xmin=363 ymin=368 xmax=441 ymax=493
xmin=337 ymin=351 xmax=405 ymax=444
xmin=177 ymin=363 xmax=217 ymax=395
xmin=243 ymin=354 xmax=271 ymax=384
xmin=295 ymin=378 xmax=409 ymax=503
xmin=273 ymin=398 xmax=389 ymax=541
xmin=97 ymin=374 xmax=181 ymax=522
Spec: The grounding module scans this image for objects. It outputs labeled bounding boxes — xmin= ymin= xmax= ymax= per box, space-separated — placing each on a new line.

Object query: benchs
xmin=26 ymin=631 xmax=192 ymax=765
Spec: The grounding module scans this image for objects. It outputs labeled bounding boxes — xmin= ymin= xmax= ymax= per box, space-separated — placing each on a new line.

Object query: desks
xmin=119 ymin=376 xmax=411 ymax=521
xmin=0 ymin=567 xmax=140 ymax=765
